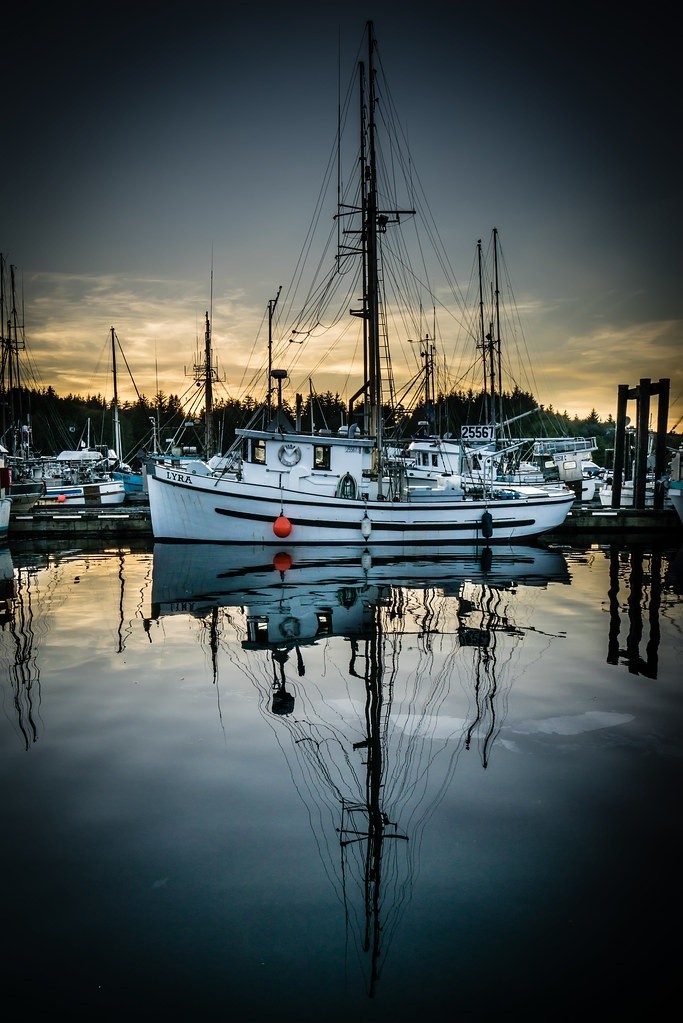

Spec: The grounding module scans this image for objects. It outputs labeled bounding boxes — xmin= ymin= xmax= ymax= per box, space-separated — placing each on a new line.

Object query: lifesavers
xmin=280 ymin=617 xmax=301 ymax=638
xmin=278 ymin=444 xmax=302 ymax=467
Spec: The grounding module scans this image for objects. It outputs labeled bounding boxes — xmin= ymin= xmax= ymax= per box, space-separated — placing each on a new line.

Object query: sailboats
xmin=0 ymin=16 xmax=683 ymax=547
